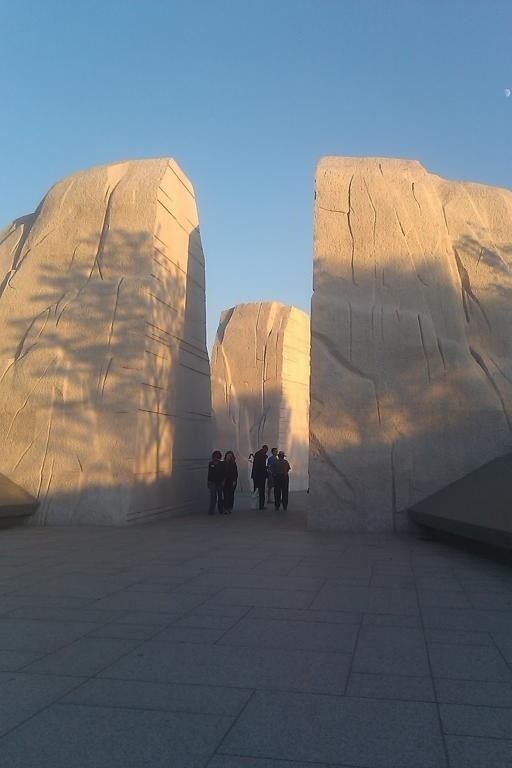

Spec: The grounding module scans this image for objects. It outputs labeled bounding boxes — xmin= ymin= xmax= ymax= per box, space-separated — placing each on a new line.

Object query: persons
xmin=207 ymin=450 xmax=224 ymax=516
xmin=253 ymin=444 xmax=269 ymax=496
xmin=252 ymin=454 xmax=269 ymax=510
xmin=215 ymin=451 xmax=238 ymax=515
xmin=267 ymin=447 xmax=278 ymax=505
xmin=271 ymin=451 xmax=292 ymax=511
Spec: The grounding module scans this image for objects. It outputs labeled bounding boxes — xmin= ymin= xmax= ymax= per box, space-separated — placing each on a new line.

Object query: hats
xmin=277 ymin=451 xmax=286 ymax=457
